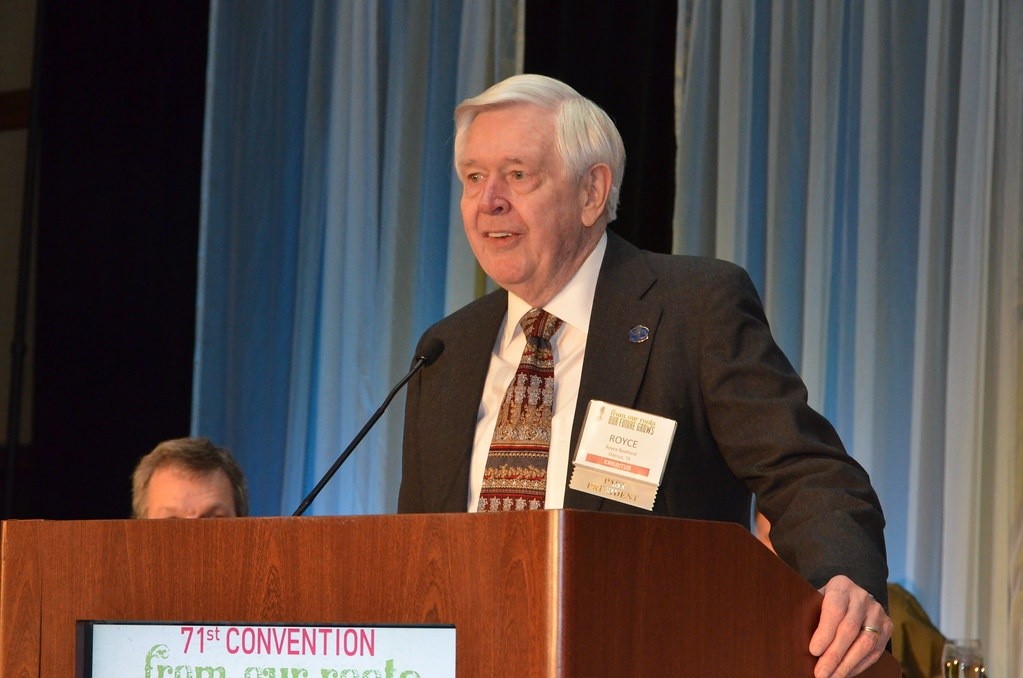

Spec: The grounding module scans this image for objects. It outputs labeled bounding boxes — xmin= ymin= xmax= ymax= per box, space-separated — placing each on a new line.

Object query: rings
xmin=861 ymin=625 xmax=881 ymax=640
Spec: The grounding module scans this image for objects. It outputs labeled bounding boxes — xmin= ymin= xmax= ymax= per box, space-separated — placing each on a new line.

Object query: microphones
xmin=292 ymin=337 xmax=446 ymax=517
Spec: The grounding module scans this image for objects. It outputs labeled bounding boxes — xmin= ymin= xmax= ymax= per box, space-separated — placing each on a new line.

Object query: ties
xmin=477 ymin=308 xmax=564 ymax=513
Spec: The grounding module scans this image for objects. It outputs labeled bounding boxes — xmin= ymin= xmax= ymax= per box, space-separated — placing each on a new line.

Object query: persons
xmin=131 ymin=439 xmax=249 ymax=519
xmin=396 ymin=73 xmax=894 ymax=678
